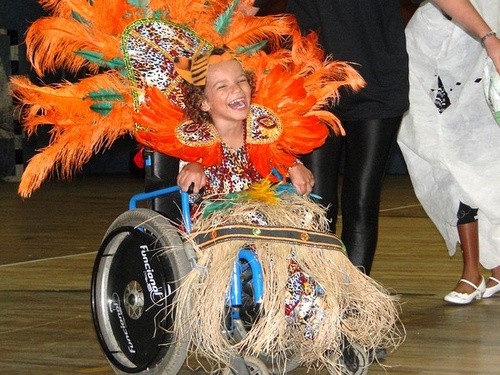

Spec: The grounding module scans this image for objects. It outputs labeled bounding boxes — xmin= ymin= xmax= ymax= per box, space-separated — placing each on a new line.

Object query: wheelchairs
xmin=89 ymin=145 xmax=369 ymax=375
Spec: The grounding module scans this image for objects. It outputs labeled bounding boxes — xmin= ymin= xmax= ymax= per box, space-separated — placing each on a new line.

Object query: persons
xmin=300 ymin=0 xmax=422 ymax=360
xmin=177 ymin=47 xmax=335 ymax=339
xmin=395 ymin=0 xmax=500 ymax=304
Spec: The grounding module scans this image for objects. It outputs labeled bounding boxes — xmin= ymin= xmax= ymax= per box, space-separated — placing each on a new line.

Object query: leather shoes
xmin=444 ymin=275 xmax=487 ymax=304
xmin=483 ymin=277 xmax=500 ymax=298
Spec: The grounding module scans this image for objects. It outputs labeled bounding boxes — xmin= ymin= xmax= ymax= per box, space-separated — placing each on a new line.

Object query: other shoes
xmin=368 ymin=343 xmax=385 ymax=359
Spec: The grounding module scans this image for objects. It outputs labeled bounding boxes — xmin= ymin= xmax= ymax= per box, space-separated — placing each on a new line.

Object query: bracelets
xmin=480 ymin=31 xmax=496 ymax=43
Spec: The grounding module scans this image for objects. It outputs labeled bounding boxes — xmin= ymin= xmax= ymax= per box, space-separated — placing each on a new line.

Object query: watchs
xmin=288 ymin=158 xmax=304 ymax=169
